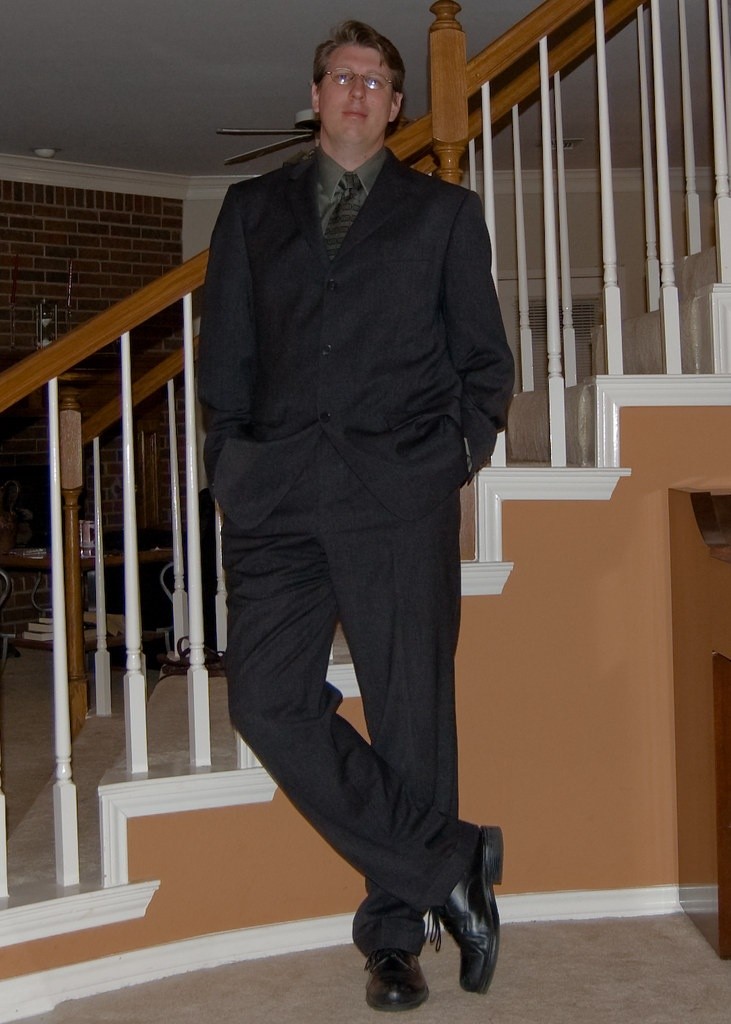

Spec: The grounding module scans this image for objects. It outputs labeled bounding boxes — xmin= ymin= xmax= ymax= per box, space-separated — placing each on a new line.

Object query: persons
xmin=200 ymin=20 xmax=518 ymax=1014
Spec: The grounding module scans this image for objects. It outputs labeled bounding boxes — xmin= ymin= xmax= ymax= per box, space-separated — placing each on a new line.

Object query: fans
xmin=216 ymin=109 xmax=319 ymax=166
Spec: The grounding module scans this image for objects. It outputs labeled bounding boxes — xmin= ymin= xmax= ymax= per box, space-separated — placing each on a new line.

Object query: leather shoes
xmin=424 ymin=824 xmax=503 ymax=995
xmin=363 ymin=944 xmax=430 ymax=1013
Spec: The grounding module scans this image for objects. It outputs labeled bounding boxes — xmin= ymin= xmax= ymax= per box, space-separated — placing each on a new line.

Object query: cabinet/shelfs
xmin=0 ymin=547 xmax=173 ymax=651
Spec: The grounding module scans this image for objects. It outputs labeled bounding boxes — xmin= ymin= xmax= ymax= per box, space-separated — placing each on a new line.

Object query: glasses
xmin=325 ymin=67 xmax=394 ymax=90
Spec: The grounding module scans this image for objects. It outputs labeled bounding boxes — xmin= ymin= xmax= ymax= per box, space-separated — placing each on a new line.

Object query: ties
xmin=323 ymin=171 xmax=362 ymax=263
